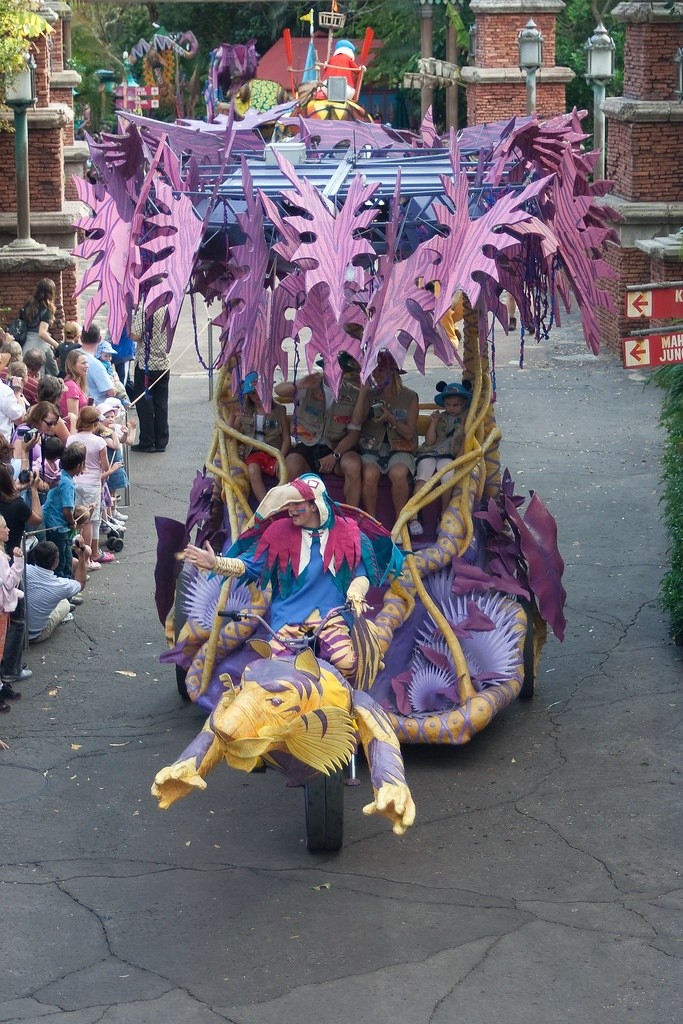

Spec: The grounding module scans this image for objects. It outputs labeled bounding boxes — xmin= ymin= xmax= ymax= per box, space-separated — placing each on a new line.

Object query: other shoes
xmin=113 ymin=512 xmax=128 ymax=520
xmin=85 ymin=562 xmax=101 ymax=571
xmin=102 ymin=521 xmax=119 ymax=530
xmin=70 ymin=604 xmax=76 ymax=610
xmin=60 ymin=613 xmax=74 ymax=623
xmin=131 ymin=445 xmax=156 ymax=452
xmin=0 ymin=683 xmax=21 ymax=699
xmin=69 ymin=597 xmax=83 ymax=604
xmin=155 ymin=446 xmax=166 ymax=451
xmin=116 ymin=524 xmax=126 ymax=531
xmin=0 ymin=703 xmax=10 ymax=712
xmin=2 ymin=669 xmax=32 ymax=682
xmin=114 ymin=519 xmax=125 ymax=525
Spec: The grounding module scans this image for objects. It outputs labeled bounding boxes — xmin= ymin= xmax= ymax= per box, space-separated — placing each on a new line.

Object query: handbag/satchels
xmin=6 ymin=308 xmax=27 ymax=346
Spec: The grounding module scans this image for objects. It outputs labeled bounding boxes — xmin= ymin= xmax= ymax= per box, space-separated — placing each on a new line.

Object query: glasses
xmin=41 ymin=418 xmax=57 ymax=426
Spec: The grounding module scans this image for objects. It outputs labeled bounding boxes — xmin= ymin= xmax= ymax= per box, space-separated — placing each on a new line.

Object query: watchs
xmin=332 ymin=452 xmax=341 ymax=461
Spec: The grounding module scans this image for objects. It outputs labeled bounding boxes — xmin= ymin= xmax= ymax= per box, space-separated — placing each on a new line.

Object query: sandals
xmin=409 ymin=519 xmax=424 ymax=535
xmin=93 ymin=552 xmax=114 ymax=562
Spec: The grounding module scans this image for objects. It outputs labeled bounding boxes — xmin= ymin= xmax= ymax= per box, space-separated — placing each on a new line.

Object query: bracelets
xmin=391 ymin=417 xmax=399 ymax=427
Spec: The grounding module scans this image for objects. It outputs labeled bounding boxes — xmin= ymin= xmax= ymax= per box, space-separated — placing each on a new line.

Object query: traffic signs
xmin=621 ymin=332 xmax=683 ymax=369
xmin=625 ymin=285 xmax=683 ymax=319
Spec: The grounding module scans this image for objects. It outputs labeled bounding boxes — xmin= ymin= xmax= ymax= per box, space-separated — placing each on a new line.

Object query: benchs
xmin=227 ymin=393 xmax=457 ymax=510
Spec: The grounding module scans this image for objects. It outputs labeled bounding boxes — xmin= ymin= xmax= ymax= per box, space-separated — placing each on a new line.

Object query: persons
xmin=408 ymin=380 xmax=473 ymax=534
xmin=151 ymin=473 xmax=415 ymax=834
xmin=351 ymin=350 xmax=419 ymax=544
xmin=130 ymin=276 xmax=171 ymax=453
xmin=19 ymin=276 xmax=59 ymax=358
xmin=224 ymin=372 xmax=291 ymax=504
xmin=0 ymin=318 xmax=131 ymax=718
xmin=274 ymin=351 xmax=361 ymax=509
xmin=105 ymin=308 xmax=136 ymax=382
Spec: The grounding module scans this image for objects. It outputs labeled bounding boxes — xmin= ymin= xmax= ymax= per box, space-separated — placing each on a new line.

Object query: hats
xmin=94 ymin=341 xmax=118 ymax=359
xmin=434 ymin=380 xmax=472 ymax=408
xmin=96 ymin=403 xmax=118 ymax=421
xmin=376 ymin=352 xmax=400 ymax=371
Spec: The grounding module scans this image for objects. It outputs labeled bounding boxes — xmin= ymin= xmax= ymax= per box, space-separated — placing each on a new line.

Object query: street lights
xmin=518 ymin=16 xmax=543 ymax=115
xmin=3 ymin=52 xmax=47 ymax=252
xmin=583 ymin=19 xmax=616 ymax=183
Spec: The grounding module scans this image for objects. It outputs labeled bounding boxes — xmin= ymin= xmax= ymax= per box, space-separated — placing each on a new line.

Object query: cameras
xmin=19 ymin=470 xmax=36 ymax=484
xmin=72 ymin=534 xmax=81 ymax=544
xmin=17 ymin=428 xmax=38 ymax=442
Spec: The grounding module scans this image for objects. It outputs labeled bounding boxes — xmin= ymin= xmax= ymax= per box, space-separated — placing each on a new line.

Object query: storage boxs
xmin=264 ymin=143 xmax=306 ymax=165
